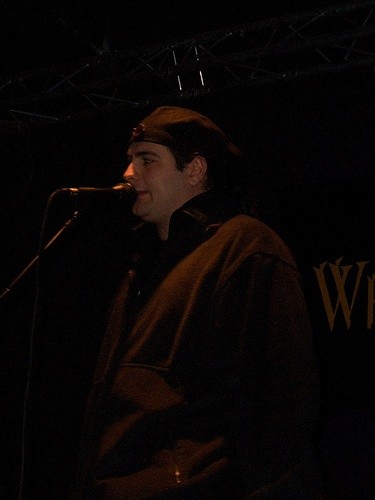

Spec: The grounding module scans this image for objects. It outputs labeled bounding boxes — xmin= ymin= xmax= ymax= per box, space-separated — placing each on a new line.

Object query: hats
xmin=128 ymin=106 xmax=243 ymax=171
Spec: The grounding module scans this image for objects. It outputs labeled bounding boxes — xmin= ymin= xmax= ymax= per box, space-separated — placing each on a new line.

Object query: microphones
xmin=63 ymin=182 xmax=138 ymax=207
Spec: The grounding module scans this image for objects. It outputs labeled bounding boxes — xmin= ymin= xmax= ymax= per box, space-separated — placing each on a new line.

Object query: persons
xmin=73 ymin=105 xmax=328 ymax=500
